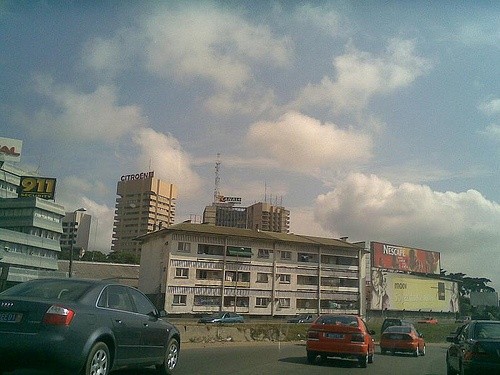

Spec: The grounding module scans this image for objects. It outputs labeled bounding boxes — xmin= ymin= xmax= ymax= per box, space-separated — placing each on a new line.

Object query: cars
xmin=287 ymin=313 xmax=318 ymax=324
xmin=419 ymin=317 xmax=438 ymax=324
xmin=0 ymin=277 xmax=181 ymax=375
xmin=380 ymin=326 xmax=427 ymax=357
xmin=197 ymin=312 xmax=245 ymax=324
xmin=446 ymin=320 xmax=500 ymax=375
xmin=306 ymin=313 xmax=376 ymax=368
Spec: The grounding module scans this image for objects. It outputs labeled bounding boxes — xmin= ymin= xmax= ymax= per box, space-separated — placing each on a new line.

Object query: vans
xmin=381 ymin=318 xmax=415 ymax=335
xmin=455 ymin=316 xmax=471 ymax=323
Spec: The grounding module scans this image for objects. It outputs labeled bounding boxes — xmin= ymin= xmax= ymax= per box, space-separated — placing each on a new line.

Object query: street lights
xmin=68 ymin=207 xmax=87 ymax=278
